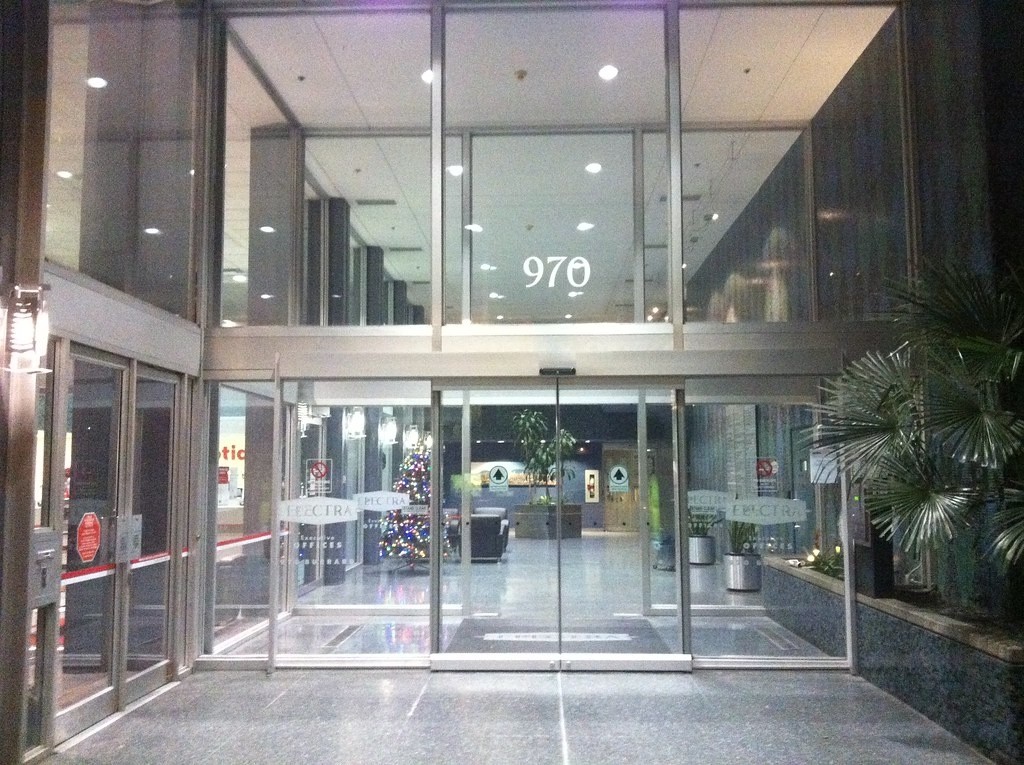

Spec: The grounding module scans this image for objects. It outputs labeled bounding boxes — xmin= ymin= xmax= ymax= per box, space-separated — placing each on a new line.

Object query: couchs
xmin=447 ymin=519 xmax=461 ymax=558
xmin=443 ymin=508 xmax=459 ymax=517
xmin=457 ymin=514 xmax=506 ymax=564
xmin=473 ymin=507 xmax=510 ymax=553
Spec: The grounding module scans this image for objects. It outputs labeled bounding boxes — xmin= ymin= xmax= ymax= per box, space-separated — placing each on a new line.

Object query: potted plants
xmin=722 ymin=492 xmax=761 ymax=591
xmin=688 ymin=508 xmax=724 ymax=565
xmin=510 ymin=408 xmax=581 ymax=540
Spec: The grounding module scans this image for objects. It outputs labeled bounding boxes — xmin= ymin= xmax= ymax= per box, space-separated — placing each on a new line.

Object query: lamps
xmin=0 ymin=276 xmax=54 ymax=377
